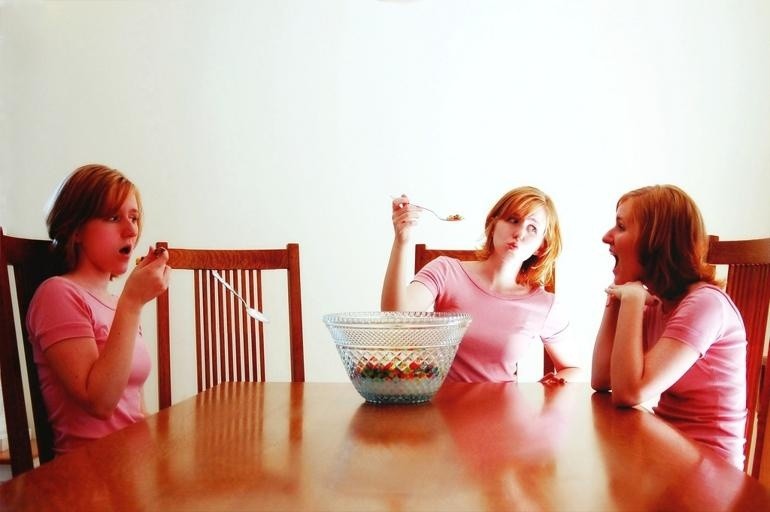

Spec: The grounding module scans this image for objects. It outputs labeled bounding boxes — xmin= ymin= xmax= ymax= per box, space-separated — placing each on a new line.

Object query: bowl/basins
xmin=325 ymin=311 xmax=471 ymax=403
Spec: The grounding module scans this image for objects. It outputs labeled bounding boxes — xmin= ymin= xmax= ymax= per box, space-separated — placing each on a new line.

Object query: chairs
xmin=0 ymin=227 xmax=74 ymax=477
xmin=414 ymin=244 xmax=556 ymax=374
xmin=156 ymin=243 xmax=305 ymax=410
xmin=705 ymin=235 xmax=770 ymax=480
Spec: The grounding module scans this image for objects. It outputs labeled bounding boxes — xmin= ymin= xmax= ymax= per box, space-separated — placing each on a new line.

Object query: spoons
xmin=211 ymin=270 xmax=272 ymax=325
xmin=405 ymin=201 xmax=465 ymax=222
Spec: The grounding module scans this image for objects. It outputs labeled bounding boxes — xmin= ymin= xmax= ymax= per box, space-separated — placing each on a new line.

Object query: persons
xmin=376 ymin=184 xmax=591 ymax=388
xmin=588 ymin=180 xmax=750 ymax=476
xmin=21 ymin=163 xmax=172 ymax=460
